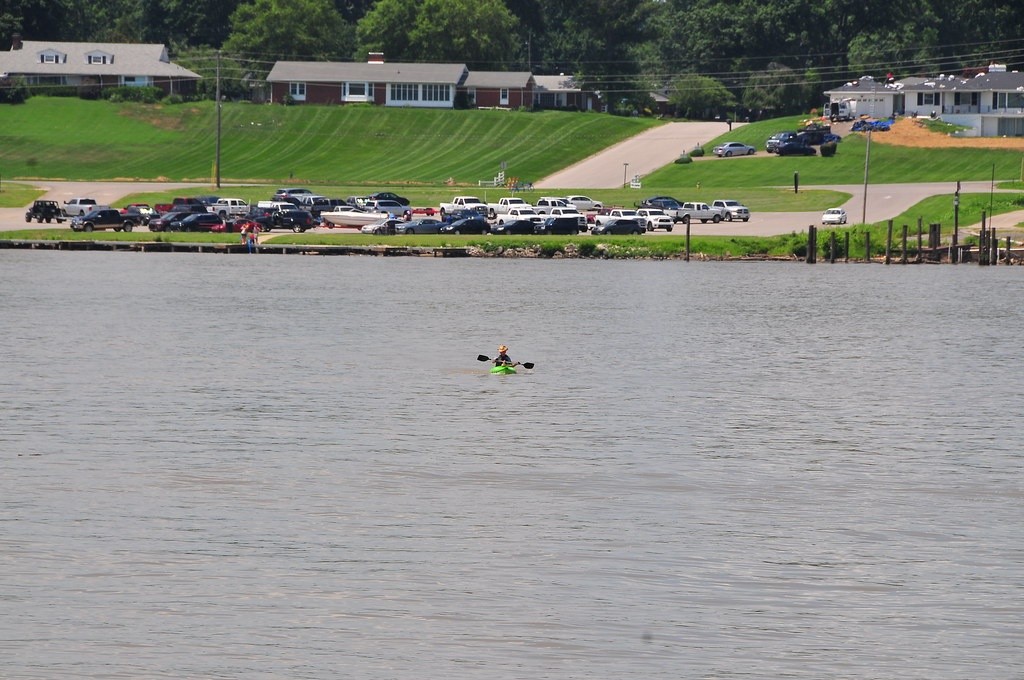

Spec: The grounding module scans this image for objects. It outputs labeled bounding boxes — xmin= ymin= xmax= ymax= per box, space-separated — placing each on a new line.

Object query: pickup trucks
xmin=539 ymin=207 xmax=588 ymax=233
xmin=487 ymin=197 xmax=533 ymax=212
xmin=439 ymin=196 xmax=487 ymax=216
xmin=595 ymin=209 xmax=648 ymax=232
xmin=70 ymin=208 xmax=141 ymax=232
xmin=662 ymin=201 xmax=723 ymax=223
xmin=61 ymin=197 xmax=111 ymax=218
xmin=705 ymin=199 xmax=750 ymax=222
xmin=496 ymin=206 xmax=539 ymax=225
xmin=531 ymin=197 xmax=576 ymax=214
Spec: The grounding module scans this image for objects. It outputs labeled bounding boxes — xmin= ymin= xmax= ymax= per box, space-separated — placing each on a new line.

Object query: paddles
xmin=477 ymin=354 xmax=534 ymax=369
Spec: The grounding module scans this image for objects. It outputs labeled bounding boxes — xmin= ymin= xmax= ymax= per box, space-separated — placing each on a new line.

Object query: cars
xmin=491 ymin=219 xmax=543 ymax=236
xmin=395 ymin=218 xmax=448 ymax=234
xmin=439 ymin=218 xmax=492 ymax=236
xmin=822 ymin=207 xmax=848 ymax=225
xmin=445 ymin=209 xmax=484 ymax=224
xmin=592 ymin=219 xmax=642 ymax=235
xmin=765 ymin=128 xmax=824 ymax=157
xmin=713 ymin=142 xmax=757 ymax=157
xmin=360 ymin=217 xmax=407 ymax=235
xmin=638 ymin=196 xmax=685 ymax=214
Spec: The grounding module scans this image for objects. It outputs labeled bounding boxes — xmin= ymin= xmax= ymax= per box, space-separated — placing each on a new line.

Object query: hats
xmin=499 ymin=345 xmax=508 ymax=352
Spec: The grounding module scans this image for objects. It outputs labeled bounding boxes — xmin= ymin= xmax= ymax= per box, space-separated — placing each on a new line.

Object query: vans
xmin=533 ymin=217 xmax=580 ymax=236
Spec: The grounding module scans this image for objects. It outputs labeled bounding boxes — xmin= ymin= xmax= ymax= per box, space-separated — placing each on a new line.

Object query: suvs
xmin=635 ymin=208 xmax=675 ymax=233
xmin=566 ymin=195 xmax=604 ymax=212
xmin=25 ymin=199 xmax=63 ymax=224
xmin=121 ymin=185 xmax=415 ymax=233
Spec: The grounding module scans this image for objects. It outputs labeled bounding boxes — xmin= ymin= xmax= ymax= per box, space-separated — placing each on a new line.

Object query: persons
xmin=492 ymin=345 xmax=519 ymax=367
xmin=388 ymin=211 xmax=394 ymax=219
xmin=406 ymin=211 xmax=412 ymax=221
xmin=240 ymin=222 xmax=261 ymax=245
xmin=264 ymin=212 xmax=271 ymax=218
xmin=446 ymin=214 xmax=453 ymax=225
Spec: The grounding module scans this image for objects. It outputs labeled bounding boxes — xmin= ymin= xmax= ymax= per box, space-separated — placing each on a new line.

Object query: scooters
xmin=911 ymin=109 xmax=937 ymax=118
